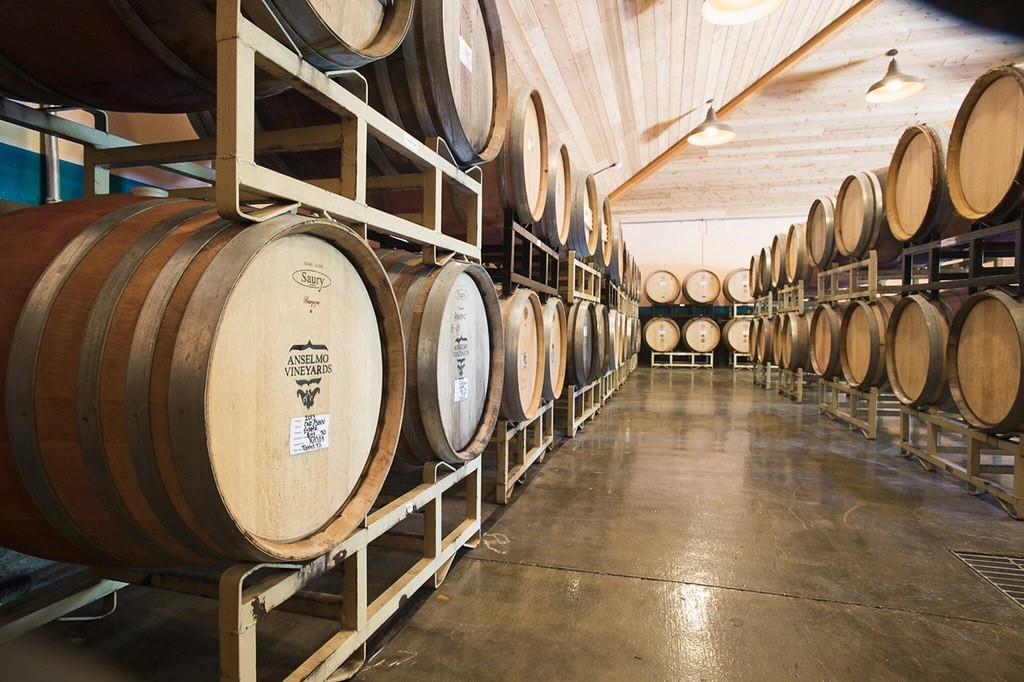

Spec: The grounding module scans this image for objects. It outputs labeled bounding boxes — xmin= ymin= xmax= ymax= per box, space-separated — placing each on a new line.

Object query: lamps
xmin=688 ymin=100 xmax=735 ymax=145
xmin=701 ymin=0 xmax=787 ymax=26
xmin=866 ymin=49 xmax=923 ymax=103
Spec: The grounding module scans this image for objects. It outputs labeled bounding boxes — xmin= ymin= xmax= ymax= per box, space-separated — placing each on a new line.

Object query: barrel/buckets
xmin=645 ymin=268 xmax=720 ymax=353
xmin=723 ymin=65 xmax=1024 ymax=430
xmin=0 ymin=0 xmax=641 ymax=600
